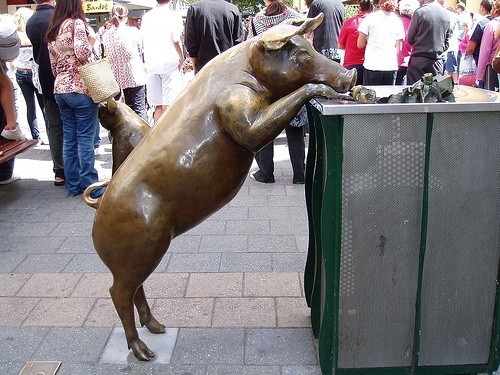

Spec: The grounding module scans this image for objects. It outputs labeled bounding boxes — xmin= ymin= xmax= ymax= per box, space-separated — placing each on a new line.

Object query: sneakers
xmin=1 ymin=122 xmax=28 ymax=140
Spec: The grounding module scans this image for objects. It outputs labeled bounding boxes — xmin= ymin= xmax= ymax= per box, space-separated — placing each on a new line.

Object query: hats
xmin=0 ymin=12 xmax=21 ymax=60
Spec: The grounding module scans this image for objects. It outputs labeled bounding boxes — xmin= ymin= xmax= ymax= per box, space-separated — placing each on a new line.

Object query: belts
xmin=18 ymin=67 xmax=32 ymax=72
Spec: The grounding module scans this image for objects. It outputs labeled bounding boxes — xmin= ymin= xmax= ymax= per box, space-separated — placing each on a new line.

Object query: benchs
xmin=0 ymin=137 xmax=39 ymax=165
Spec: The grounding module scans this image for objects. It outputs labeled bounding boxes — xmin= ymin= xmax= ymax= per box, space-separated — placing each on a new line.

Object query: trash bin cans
xmin=303 ymin=84 xmax=500 ymax=375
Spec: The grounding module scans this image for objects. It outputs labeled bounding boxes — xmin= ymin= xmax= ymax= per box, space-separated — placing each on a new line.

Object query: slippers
xmin=54 ymin=173 xmax=65 ymax=185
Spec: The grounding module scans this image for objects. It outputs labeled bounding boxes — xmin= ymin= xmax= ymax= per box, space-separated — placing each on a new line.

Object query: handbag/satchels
xmin=78 ymin=57 xmax=121 ymax=104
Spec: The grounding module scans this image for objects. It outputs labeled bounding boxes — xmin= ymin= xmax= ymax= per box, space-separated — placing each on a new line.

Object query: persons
xmin=0 ymin=1 xmax=500 ymax=199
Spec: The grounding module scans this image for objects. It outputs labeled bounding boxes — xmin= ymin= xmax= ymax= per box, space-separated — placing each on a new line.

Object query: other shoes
xmin=250 ymin=171 xmax=257 ymax=179
xmin=0 ymin=176 xmax=21 ymax=184
xmin=94 ymin=146 xmax=105 ymax=155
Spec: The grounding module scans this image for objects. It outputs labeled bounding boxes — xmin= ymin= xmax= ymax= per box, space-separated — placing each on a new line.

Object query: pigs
xmin=96 ymin=85 xmax=153 ymax=179
xmin=82 ymin=12 xmax=357 ymax=363
